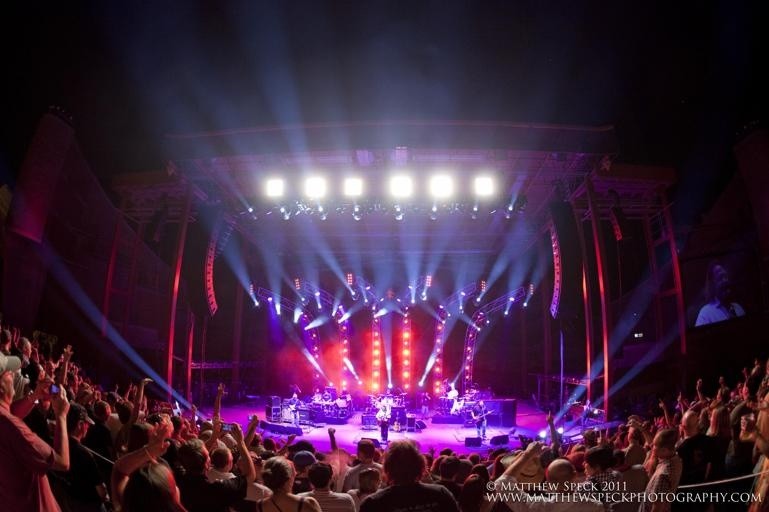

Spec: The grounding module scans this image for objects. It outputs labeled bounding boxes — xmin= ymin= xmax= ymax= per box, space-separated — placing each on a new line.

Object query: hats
xmin=500 ymin=452 xmax=546 ymax=487
xmin=94 ymin=398 xmax=136 ymax=423
xmin=294 ymin=450 xmax=318 ymax=465
xmin=0 ymin=350 xmax=21 ymax=373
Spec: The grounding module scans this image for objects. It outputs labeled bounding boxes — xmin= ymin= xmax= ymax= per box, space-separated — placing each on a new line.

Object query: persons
xmin=693 ymin=261 xmax=745 ymax=328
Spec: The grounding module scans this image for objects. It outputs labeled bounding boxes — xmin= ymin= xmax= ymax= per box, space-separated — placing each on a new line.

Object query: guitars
xmin=474 ymin=410 xmax=494 ymax=423
xmin=394 ymin=411 xmax=400 ymax=432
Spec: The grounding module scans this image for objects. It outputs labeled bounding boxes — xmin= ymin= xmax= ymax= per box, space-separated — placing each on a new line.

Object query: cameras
xmin=48 ymin=384 xmax=60 ymax=394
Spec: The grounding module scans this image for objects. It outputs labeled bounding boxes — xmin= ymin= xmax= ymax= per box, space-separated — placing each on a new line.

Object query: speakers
xmin=485 ymin=414 xmax=502 ymax=428
xmin=362 ymin=415 xmax=377 ymax=425
xmin=483 ymin=399 xmax=503 ymax=415
xmin=502 ymin=399 xmax=516 ymax=427
xmin=416 ymin=420 xmax=427 ymax=429
xmin=268 ymin=396 xmax=280 ymax=406
xmin=465 ymin=437 xmax=481 ymax=447
xmin=490 ymin=435 xmax=509 ymax=445
xmin=361 ymin=437 xmax=381 ymax=448
